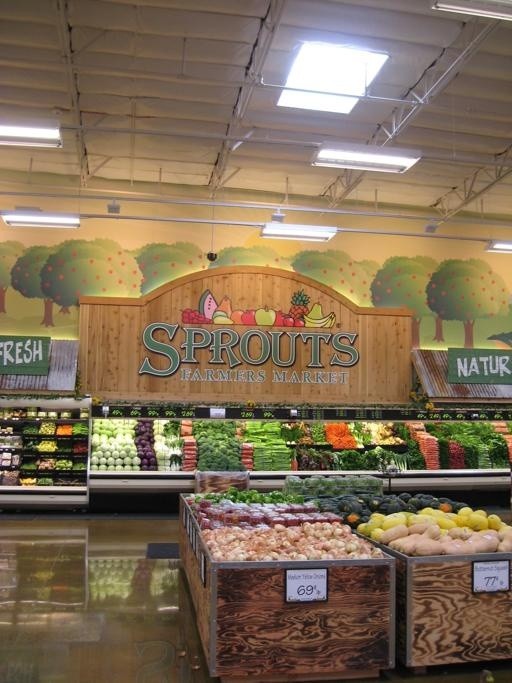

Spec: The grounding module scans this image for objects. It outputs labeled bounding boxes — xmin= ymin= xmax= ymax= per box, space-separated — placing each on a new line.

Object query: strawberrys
xmin=283 ymin=318 xmax=294 ymax=326
xmin=294 ymin=319 xmax=305 ymax=327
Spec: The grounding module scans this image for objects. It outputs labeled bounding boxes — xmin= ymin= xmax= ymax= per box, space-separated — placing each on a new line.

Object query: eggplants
xmin=320 ymin=454 xmax=334 ymax=470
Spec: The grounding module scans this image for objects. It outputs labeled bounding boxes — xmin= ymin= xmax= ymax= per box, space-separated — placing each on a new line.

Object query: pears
xmin=214 ymin=296 xmax=232 ymax=318
xmin=307 ymin=302 xmax=323 ymax=319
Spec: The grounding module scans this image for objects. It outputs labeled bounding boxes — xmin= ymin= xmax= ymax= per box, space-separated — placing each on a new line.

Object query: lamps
xmin=1 ymin=208 xmax=81 ymax=229
xmin=105 ymin=195 xmax=440 ymax=235
xmin=260 ymin=220 xmax=338 ymax=243
xmin=431 ymin=0 xmax=512 ymax=23
xmin=485 ymin=239 xmax=512 ymax=255
xmin=310 ymin=138 xmax=423 ymax=174
xmin=0 ymin=117 xmax=63 ymax=148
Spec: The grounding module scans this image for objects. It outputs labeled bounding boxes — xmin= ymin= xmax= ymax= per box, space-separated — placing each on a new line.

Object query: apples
xmin=241 ymin=311 xmax=257 ymax=326
xmin=214 ymin=310 xmax=228 ymax=317
xmin=255 ymin=305 xmax=276 ymax=326
xmin=273 ymin=311 xmax=285 ymax=327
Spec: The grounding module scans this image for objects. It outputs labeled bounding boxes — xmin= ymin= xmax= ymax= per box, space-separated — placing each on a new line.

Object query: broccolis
xmin=193 ymin=420 xmax=246 ymax=471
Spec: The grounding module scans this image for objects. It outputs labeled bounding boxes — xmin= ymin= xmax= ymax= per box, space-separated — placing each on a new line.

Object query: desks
xmin=178 ymin=489 xmax=398 ymax=682
xmin=348 ymin=526 xmax=512 ymax=676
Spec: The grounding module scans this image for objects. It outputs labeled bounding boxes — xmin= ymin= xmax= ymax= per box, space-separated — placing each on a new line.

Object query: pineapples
xmin=288 ymin=290 xmax=311 ymax=321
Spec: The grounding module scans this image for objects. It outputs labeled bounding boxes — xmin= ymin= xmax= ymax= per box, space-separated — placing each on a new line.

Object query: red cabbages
xmin=131 ymin=559 xmax=151 ymax=594
xmin=134 ymin=419 xmax=159 ymax=471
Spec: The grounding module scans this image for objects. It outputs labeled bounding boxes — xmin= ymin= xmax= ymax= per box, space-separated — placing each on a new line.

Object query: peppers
xmin=195 ymin=487 xmax=304 ymax=505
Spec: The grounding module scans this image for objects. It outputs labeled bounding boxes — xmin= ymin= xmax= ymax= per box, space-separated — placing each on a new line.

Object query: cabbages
xmin=87 ymin=559 xmax=138 ymax=600
xmin=154 ymin=418 xmax=170 ymax=471
xmin=91 ymin=418 xmax=141 ymax=471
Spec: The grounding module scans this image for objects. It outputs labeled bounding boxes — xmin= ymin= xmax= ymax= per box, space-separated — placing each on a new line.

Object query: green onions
xmin=393 ymin=454 xmax=407 ymax=471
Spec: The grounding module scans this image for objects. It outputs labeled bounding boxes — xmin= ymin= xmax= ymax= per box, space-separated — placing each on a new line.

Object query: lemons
xmin=214 ymin=317 xmax=235 ymax=325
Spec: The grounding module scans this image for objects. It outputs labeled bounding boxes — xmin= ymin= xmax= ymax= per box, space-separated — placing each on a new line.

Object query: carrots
xmin=493 ymin=423 xmax=512 ymax=466
xmin=235 ymin=427 xmax=254 ymax=471
xmin=180 ymin=420 xmax=197 ymax=471
xmin=405 ymin=423 xmax=440 ymax=470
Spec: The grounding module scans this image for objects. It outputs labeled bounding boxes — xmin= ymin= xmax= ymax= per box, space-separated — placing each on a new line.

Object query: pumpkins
xmin=304 ymin=493 xmax=512 ymax=556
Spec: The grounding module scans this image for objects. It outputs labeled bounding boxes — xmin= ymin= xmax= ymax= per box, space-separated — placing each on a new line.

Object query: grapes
xmin=181 ymin=308 xmax=213 ymax=324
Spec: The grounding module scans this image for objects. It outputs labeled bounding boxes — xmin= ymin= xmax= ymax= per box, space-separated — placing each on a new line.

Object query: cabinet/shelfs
xmin=0 ymin=392 xmax=92 ymax=513
xmin=1 ymin=514 xmax=89 ymax=611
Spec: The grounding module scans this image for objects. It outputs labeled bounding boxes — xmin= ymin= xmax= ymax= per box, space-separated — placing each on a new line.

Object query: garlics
xmin=202 ymin=522 xmax=389 ymax=562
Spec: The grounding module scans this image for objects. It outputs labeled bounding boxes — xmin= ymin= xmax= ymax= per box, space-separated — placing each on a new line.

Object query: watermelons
xmin=199 ymin=288 xmax=218 ymax=319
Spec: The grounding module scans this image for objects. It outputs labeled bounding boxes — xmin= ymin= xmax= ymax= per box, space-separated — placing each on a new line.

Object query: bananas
xmin=303 ymin=312 xmax=336 ymax=328
